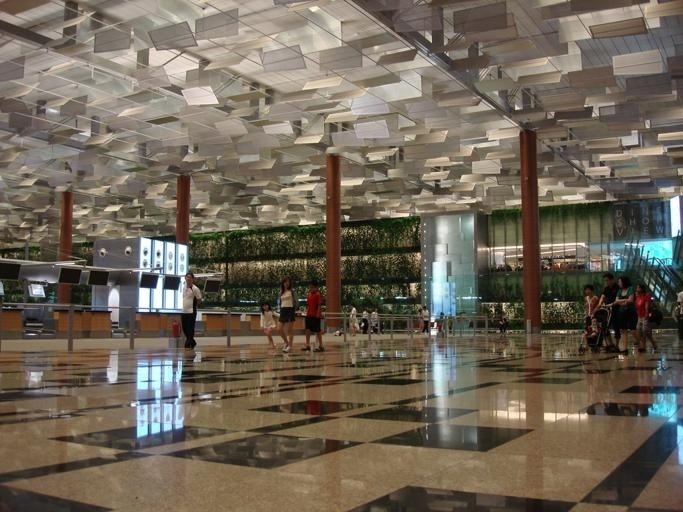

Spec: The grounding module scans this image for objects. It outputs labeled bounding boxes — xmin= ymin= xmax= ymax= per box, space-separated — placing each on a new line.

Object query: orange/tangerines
xmin=579 ymin=306 xmax=617 ymax=353
xmin=373 ymin=315 xmax=384 ymax=334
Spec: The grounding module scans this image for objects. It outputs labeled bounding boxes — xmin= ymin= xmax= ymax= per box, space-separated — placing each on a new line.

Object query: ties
xmin=87 ymin=269 xmax=110 ymax=287
xmin=58 ymin=266 xmax=82 ymax=286
xmin=162 ymin=275 xmax=181 ymax=290
xmin=203 ymin=278 xmax=221 ymax=293
xmin=139 ymin=271 xmax=159 ymax=289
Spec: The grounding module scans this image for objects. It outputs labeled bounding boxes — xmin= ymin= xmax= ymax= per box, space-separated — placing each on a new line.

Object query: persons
xmin=499 ymin=318 xmax=507 ymax=332
xmin=547 ymin=259 xmax=560 ymax=269
xmin=578 ymin=272 xmax=657 ymax=354
xmin=417 ymin=306 xmax=453 ymax=335
xmin=344 ymin=304 xmax=378 ymax=336
xmin=260 ymin=301 xmax=280 ymax=349
xmin=276 ymin=275 xmax=297 ymax=352
xmin=302 ymin=279 xmax=325 ymax=352
xmin=582 ymin=360 xmax=652 ymax=416
xmin=677 ymin=281 xmax=682 ymax=338
xmin=181 ymin=272 xmax=202 ymax=349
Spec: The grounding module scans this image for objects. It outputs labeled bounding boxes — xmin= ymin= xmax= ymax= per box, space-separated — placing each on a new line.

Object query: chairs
xmin=301 ymin=346 xmax=311 ymax=351
xmin=648 ymin=308 xmax=662 ymax=323
xmin=654 ymin=345 xmax=658 ymax=350
xmin=313 ymin=346 xmax=324 ymax=352
xmin=618 ymin=349 xmax=628 ymax=354
xmin=282 ymin=343 xmax=291 ymax=353
xmin=638 ymin=348 xmax=645 ymax=352
